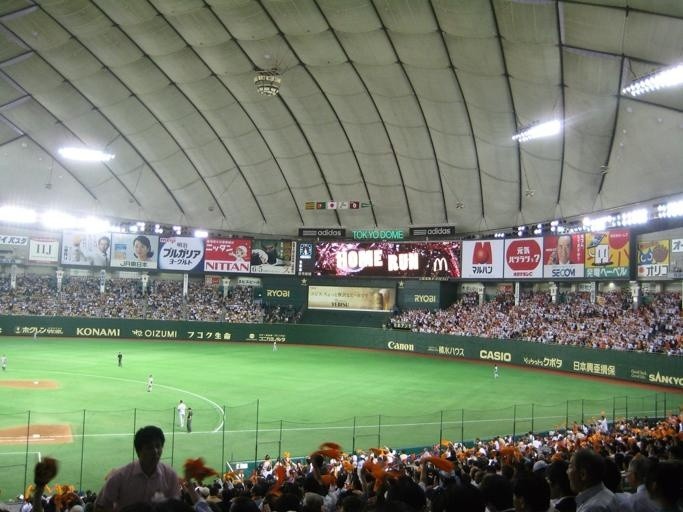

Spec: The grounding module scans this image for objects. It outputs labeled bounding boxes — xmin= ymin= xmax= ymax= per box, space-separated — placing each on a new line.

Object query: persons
xmin=1 ymin=353 xmax=7 ymax=370
xmin=547 ymin=235 xmax=576 ymax=265
xmin=493 ymin=362 xmax=499 ymax=379
xmin=132 ymin=235 xmax=154 ymax=262
xmin=273 ymin=338 xmax=276 ymax=351
xmin=0 ymin=409 xmax=683 ymax=512
xmin=258 ymin=240 xmax=289 ymax=266
xmin=82 ymin=236 xmax=110 ymax=268
xmin=226 ymin=243 xmax=248 ymax=263
xmin=146 ymin=373 xmax=153 ymax=392
xmin=381 ymin=278 xmax=683 ymax=361
xmin=116 ymin=351 xmax=122 ymax=368
xmin=175 ymin=399 xmax=187 ymax=429
xmin=1 ymin=265 xmax=303 ymax=324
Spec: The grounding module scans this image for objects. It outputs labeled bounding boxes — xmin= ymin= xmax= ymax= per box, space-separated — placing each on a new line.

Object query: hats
xmin=532 ymin=460 xmax=552 ymax=473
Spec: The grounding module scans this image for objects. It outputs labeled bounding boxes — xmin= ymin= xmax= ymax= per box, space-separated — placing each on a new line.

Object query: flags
xmin=304 ymin=200 xmax=316 ymax=210
xmin=326 ymin=202 xmax=336 ymax=210
xmin=316 ymin=202 xmax=326 ymax=211
xmin=337 ymin=201 xmax=348 ymax=210
xmin=349 ymin=201 xmax=359 ymax=209
